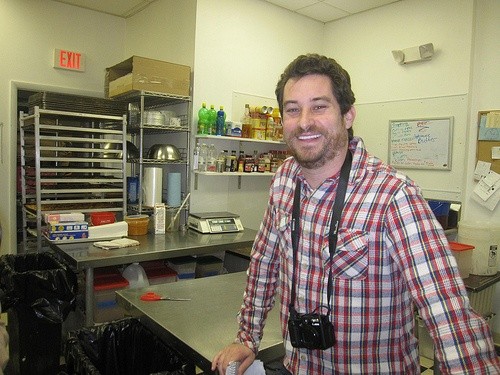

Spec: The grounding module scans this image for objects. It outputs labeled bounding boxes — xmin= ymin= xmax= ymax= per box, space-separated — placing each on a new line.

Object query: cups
xmin=166 ymin=210 xmax=180 ymax=232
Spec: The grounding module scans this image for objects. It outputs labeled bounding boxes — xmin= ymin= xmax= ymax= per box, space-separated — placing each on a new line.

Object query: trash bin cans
xmin=67 ymin=317 xmax=196 ymax=375
xmin=0 ymin=252 xmax=77 ymax=375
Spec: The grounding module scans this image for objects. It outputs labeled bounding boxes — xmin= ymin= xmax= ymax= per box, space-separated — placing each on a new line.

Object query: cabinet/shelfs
xmin=20 ymin=105 xmax=127 ymax=252
xmin=102 ymin=90 xmax=192 ymax=223
xmin=192 ymin=135 xmax=287 ymax=177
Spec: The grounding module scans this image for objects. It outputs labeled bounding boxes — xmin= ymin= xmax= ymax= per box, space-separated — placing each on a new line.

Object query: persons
xmin=212 ymin=54 xmax=500 ymax=375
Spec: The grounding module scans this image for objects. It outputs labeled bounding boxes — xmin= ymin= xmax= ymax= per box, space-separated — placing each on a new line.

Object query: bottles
xmin=193 ymin=143 xmax=294 ymax=171
xmin=198 ymin=102 xmax=226 ymax=136
xmin=240 ymin=104 xmax=252 ymax=137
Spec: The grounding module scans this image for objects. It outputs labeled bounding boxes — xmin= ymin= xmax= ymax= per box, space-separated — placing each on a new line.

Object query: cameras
xmin=288 ymin=313 xmax=334 ymax=350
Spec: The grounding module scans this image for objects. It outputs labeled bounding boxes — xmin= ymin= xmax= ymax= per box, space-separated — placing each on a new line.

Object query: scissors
xmin=141 ymin=292 xmax=190 ymax=301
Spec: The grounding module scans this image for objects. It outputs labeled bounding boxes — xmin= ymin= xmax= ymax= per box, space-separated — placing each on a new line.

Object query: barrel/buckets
xmin=89 ymin=212 xmax=116 ymax=225
xmin=458 ymin=221 xmax=500 ymax=276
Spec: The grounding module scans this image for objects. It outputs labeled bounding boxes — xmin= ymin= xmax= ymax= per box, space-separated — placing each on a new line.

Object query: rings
xmin=228 ymin=361 xmax=233 ymax=365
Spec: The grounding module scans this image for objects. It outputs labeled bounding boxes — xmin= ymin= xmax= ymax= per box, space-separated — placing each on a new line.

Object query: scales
xmin=187 ymin=211 xmax=245 ymax=233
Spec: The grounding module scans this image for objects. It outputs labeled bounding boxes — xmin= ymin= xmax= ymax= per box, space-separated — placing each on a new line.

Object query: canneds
xmin=248 ymin=105 xmax=275 ymax=141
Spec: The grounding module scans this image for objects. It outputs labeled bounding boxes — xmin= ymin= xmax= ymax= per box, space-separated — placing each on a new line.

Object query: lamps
xmin=392 ymin=43 xmax=434 ymax=64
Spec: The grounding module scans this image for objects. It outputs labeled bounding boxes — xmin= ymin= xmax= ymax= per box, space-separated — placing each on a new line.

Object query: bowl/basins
xmin=149 ymin=144 xmax=181 ymax=160
xmin=125 ymin=215 xmax=151 ymax=236
xmin=139 ymin=109 xmax=176 ymax=127
xmin=101 ymin=140 xmax=140 ymax=160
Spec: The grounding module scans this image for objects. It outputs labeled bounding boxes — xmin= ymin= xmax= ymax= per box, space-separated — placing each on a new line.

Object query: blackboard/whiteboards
xmin=388 ymin=116 xmax=454 ymax=171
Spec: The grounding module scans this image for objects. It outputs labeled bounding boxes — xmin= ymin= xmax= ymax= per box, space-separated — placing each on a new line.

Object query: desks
xmin=115 ymin=272 xmax=286 ymax=374
xmin=44 ymin=228 xmax=258 ymax=327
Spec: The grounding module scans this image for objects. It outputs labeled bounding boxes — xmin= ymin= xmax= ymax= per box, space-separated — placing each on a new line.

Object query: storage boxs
xmin=418 ymin=242 xmax=500 ymax=359
xmin=104 ymin=55 xmax=190 ymax=99
xmin=93 ymin=257 xmax=223 ymax=322
xmin=45 ymin=211 xmax=89 ymax=241
